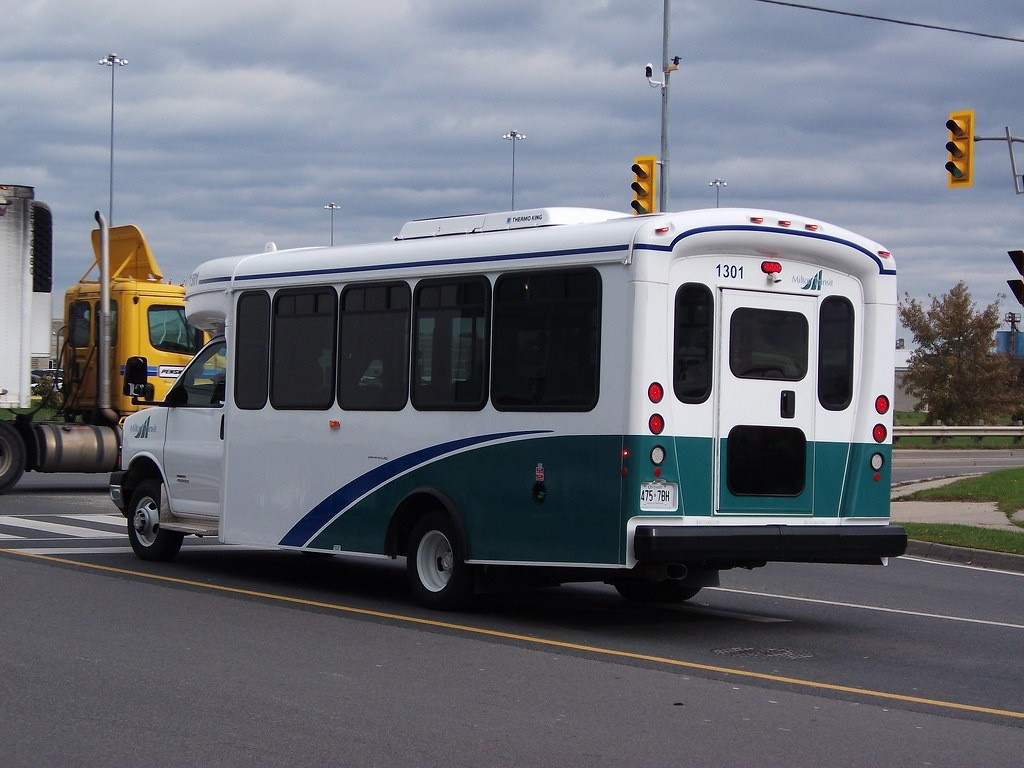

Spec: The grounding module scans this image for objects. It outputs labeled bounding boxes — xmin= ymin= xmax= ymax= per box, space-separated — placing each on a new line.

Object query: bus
xmin=108 ymin=205 xmax=908 ymax=613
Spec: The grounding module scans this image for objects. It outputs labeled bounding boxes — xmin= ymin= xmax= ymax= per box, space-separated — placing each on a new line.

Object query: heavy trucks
xmin=0 ymin=184 xmax=227 ymax=495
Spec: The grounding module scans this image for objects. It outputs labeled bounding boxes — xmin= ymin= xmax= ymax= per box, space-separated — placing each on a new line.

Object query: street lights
xmin=502 ymin=131 xmax=527 ymax=211
xmin=710 ymin=178 xmax=727 ymax=206
xmin=99 ymin=53 xmax=130 ymax=228
xmin=324 ymin=202 xmax=341 ymax=246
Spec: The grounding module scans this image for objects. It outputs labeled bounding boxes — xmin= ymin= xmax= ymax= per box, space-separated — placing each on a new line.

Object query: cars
xmin=31 ymin=369 xmax=63 ymax=393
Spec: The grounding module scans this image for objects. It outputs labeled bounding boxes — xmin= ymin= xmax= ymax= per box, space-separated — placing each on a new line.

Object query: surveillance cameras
xmin=645 ymin=63 xmax=653 ymax=71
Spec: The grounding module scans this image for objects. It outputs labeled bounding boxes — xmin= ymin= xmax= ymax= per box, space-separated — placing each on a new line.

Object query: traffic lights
xmin=1005 ymin=248 xmax=1024 ymax=307
xmin=631 ymin=156 xmax=655 ymax=215
xmin=945 ymin=110 xmax=973 ymax=187
